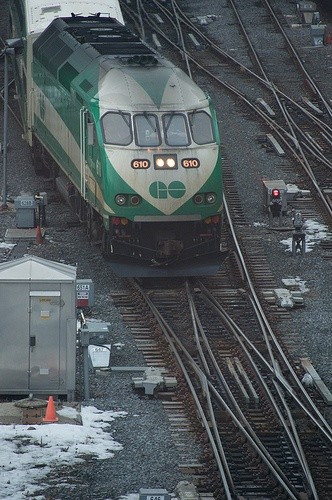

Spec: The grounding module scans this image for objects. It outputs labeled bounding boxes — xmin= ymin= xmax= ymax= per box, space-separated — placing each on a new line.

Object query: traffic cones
xmin=43 ymin=396 xmax=59 ymax=423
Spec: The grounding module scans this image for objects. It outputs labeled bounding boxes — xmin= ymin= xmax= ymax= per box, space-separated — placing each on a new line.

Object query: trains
xmin=18 ymin=0 xmax=225 ymax=264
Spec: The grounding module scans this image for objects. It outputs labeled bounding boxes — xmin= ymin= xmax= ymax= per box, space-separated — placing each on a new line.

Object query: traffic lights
xmin=272 ymin=189 xmax=281 ymax=200
